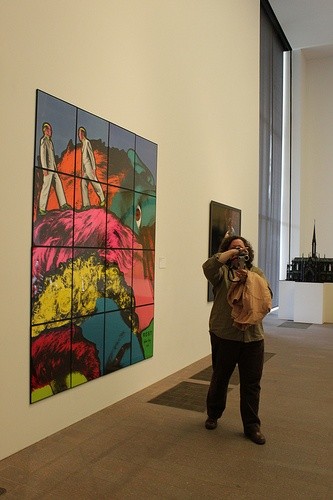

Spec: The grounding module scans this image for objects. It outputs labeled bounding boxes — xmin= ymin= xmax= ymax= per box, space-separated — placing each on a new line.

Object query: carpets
xmin=278 ymin=321 xmax=312 ymax=329
xmin=190 ymin=364 xmax=241 ymax=386
xmin=263 ymin=352 xmax=275 ymax=364
xmin=147 ymin=381 xmax=234 ymax=413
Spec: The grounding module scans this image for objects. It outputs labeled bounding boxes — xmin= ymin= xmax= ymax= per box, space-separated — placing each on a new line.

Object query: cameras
xmin=237 ymin=252 xmax=249 ymax=264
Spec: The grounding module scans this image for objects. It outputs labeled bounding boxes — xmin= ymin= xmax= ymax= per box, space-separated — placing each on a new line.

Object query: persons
xmin=202 ymin=235 xmax=273 ymax=445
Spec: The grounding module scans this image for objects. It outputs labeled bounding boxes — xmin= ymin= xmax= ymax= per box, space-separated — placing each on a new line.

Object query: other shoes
xmin=244 ymin=429 xmax=266 ymax=444
xmin=205 ymin=416 xmax=218 ymax=430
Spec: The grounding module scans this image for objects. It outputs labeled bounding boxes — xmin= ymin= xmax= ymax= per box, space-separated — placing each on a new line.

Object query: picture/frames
xmin=207 ymin=200 xmax=242 ymax=302
